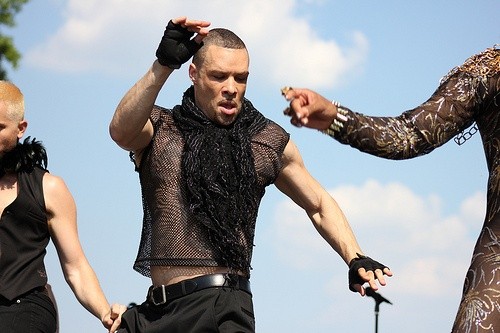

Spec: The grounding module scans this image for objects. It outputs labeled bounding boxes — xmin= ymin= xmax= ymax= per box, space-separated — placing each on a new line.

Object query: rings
xmin=281 ymin=85 xmax=299 ymax=98
xmin=284 ymin=107 xmax=294 ymax=116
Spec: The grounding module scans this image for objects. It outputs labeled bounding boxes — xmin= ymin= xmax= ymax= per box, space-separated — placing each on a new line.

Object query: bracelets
xmin=317 ymin=99 xmax=349 ymax=137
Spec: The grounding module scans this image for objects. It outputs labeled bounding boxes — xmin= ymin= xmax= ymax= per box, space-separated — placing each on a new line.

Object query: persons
xmin=110 ymin=17 xmax=393 ymax=333
xmin=0 ymin=80 xmax=127 ymax=333
xmin=281 ymin=44 xmax=500 ymax=333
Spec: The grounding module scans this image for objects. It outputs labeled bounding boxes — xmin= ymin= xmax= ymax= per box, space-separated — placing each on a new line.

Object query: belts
xmin=147 ymin=273 xmax=253 ymax=306
